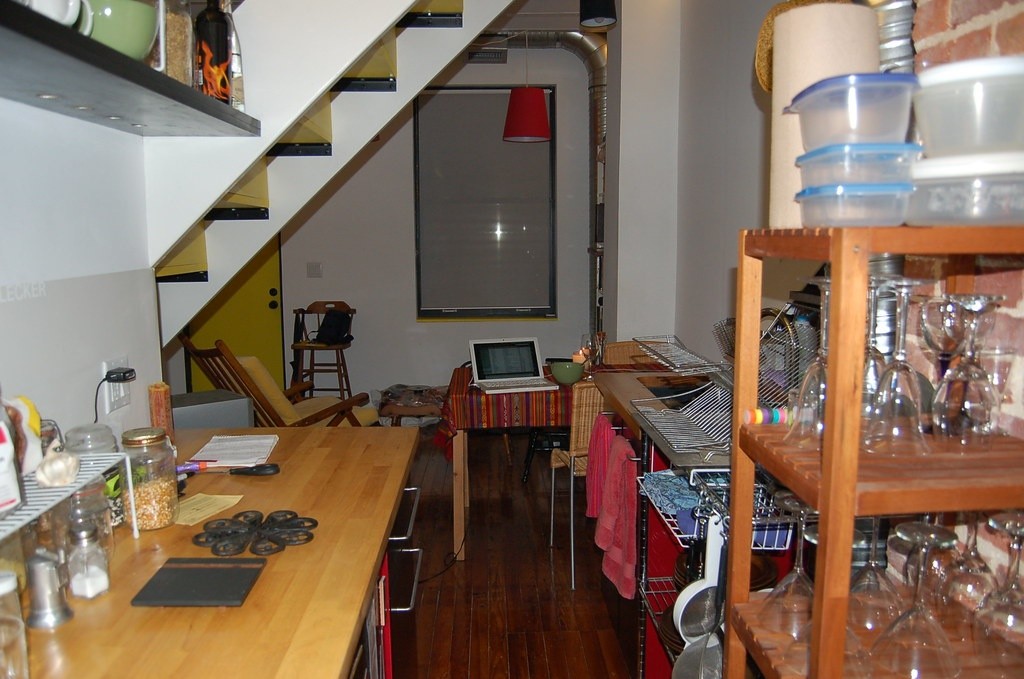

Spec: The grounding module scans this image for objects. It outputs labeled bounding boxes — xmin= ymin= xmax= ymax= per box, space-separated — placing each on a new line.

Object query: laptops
xmin=469 ymin=337 xmax=559 ymax=394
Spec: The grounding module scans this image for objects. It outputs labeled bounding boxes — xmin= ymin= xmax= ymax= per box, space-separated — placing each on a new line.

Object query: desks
xmin=432 ymin=362 xmax=714 ymax=560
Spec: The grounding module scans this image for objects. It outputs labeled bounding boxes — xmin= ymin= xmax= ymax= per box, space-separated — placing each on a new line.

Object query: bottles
xmin=63 ymin=425 xmax=123 ymax=527
xmin=190 ymin=0 xmax=233 ymax=105
xmin=1 ymin=474 xmax=116 ymax=679
xmin=146 ymin=0 xmax=193 ymax=87
xmin=119 ymin=427 xmax=180 ymax=529
xmin=790 ymin=314 xmax=817 ymax=387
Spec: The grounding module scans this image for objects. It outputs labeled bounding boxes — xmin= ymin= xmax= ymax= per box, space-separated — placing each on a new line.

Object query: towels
xmin=585 ymin=414 xmax=638 ymax=600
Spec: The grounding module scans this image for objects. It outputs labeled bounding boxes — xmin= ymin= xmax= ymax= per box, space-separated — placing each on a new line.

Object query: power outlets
xmin=103 ymin=358 xmax=130 ymax=414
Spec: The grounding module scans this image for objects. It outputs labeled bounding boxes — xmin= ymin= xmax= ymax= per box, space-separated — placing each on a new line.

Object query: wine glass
xmin=758 ymin=496 xmax=1023 ymax=677
xmin=581 ymin=333 xmax=599 ymax=382
xmin=780 ymin=274 xmax=1007 ymax=458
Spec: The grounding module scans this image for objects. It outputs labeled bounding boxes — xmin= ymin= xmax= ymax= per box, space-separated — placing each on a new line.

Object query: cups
xmin=572 ymin=351 xmax=586 ymax=363
xmin=15 ymin=0 xmax=81 ymax=26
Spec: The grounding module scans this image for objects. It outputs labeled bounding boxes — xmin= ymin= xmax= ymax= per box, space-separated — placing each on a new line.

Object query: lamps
xmin=502 ymin=31 xmax=551 ymax=142
xmin=579 ymin=0 xmax=617 ymax=27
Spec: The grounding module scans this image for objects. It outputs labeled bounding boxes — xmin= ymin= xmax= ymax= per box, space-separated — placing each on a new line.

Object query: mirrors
xmin=412 ymin=83 xmax=558 ymax=323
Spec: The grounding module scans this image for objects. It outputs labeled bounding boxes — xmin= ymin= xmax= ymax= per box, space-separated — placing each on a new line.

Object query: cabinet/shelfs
xmin=380 ymin=472 xmax=423 ymax=679
xmin=634 ymin=224 xmax=1023 ymax=679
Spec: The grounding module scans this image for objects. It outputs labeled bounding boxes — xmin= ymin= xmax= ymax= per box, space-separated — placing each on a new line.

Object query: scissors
xmin=195 ymin=464 xmax=280 ymax=476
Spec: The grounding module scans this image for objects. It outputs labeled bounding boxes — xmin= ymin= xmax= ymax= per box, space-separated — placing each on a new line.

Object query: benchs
xmin=379 ymin=398 xmax=443 ymax=426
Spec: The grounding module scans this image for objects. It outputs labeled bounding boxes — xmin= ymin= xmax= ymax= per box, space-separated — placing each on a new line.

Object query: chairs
xmin=521 ymin=358 xmax=573 ymax=486
xmin=603 ymin=341 xmax=666 ymax=364
xmin=550 ymin=383 xmax=614 ymax=591
xmin=178 ymin=334 xmax=383 ymax=427
xmin=461 ymin=360 xmax=515 ymax=467
xmin=291 ymin=300 xmax=357 ymax=400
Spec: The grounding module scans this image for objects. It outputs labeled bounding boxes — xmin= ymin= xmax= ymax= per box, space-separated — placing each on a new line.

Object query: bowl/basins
xmin=75 ymin=0 xmax=158 ymax=61
xmin=551 ymin=362 xmax=585 ymax=385
xmin=780 ymin=55 xmax=1024 ymax=228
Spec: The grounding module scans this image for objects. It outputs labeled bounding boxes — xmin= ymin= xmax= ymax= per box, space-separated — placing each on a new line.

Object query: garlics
xmin=35 ymin=438 xmax=81 ymax=486
